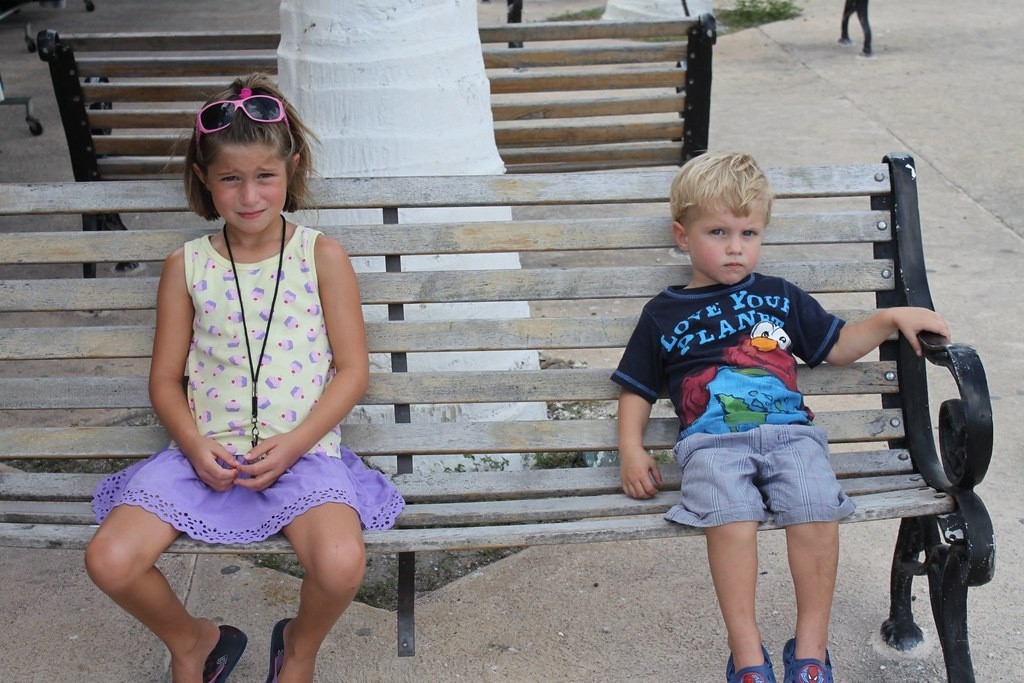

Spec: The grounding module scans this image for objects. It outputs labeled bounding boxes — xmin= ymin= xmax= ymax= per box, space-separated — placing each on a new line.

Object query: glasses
xmin=194 ymin=94 xmax=291 ymax=163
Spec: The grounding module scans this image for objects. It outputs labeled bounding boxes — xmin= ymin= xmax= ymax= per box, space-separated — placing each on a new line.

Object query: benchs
xmin=34 ymin=13 xmax=722 ymax=300
xmin=0 ymin=151 xmax=1000 ymax=683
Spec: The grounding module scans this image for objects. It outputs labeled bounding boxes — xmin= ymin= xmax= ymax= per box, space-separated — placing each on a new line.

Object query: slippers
xmin=202 ymin=625 xmax=247 ymax=683
xmin=264 ymin=617 xmax=293 ymax=683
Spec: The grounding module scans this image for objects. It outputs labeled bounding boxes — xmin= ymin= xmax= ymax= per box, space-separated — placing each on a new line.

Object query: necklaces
xmin=222 ymin=213 xmax=287 ymax=464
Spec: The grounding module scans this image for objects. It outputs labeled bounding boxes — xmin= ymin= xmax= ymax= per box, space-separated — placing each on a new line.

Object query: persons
xmin=83 ymin=71 xmax=406 ymax=683
xmin=610 ymin=150 xmax=950 ymax=683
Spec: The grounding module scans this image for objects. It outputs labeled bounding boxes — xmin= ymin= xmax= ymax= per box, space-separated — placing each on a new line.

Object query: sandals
xmin=726 ymin=643 xmax=777 ymax=683
xmin=783 ymin=637 xmax=834 ymax=683
